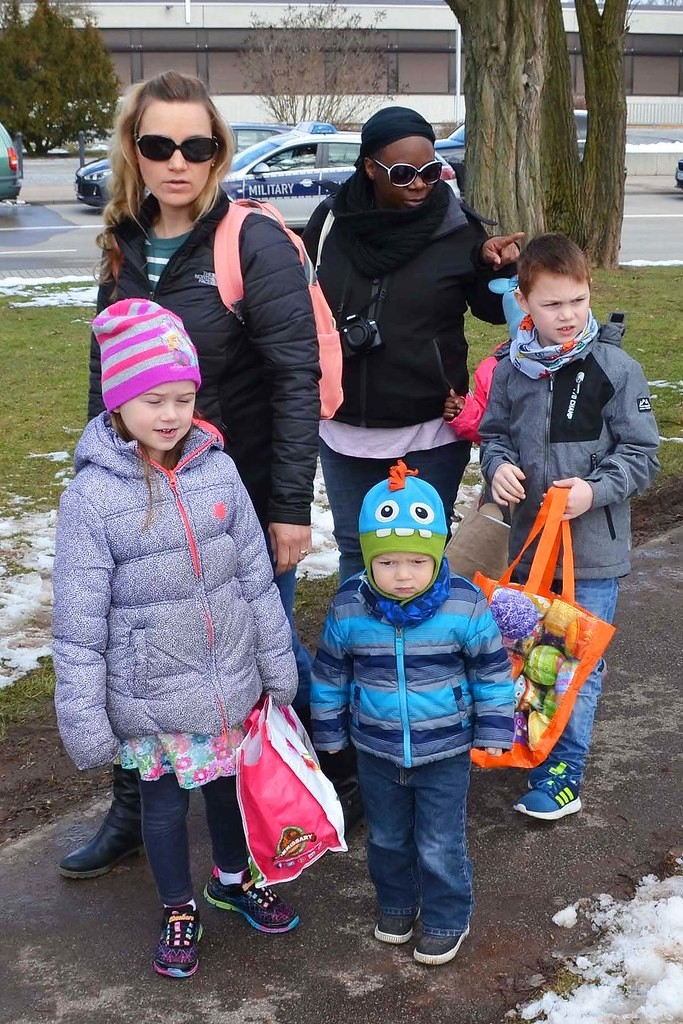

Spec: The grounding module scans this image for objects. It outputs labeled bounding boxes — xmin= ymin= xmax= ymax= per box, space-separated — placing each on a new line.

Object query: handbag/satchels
xmin=469 ymin=485 xmax=618 ymax=769
xmin=444 ymin=506 xmax=510 ymax=583
xmin=233 ymin=696 xmax=350 ymax=887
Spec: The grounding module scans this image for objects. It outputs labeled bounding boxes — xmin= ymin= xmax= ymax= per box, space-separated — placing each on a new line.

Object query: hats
xmin=359 ymin=107 xmax=438 ymax=163
xmin=488 ymin=274 xmax=529 ymax=342
xmin=92 ymin=297 xmax=201 ymax=416
xmin=359 ymin=460 xmax=449 ymax=603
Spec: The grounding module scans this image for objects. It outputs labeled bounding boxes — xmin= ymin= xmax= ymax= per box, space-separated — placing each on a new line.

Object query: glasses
xmin=368 ymin=154 xmax=444 ymax=189
xmin=133 ymin=132 xmax=219 ymax=163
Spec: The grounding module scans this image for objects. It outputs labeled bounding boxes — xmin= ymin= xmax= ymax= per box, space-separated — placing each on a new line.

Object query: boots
xmin=58 ymin=762 xmax=143 ymax=877
xmin=290 ymin=699 xmax=360 ymax=799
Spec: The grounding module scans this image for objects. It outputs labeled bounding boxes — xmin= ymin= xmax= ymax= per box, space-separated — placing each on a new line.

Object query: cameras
xmin=339 ymin=313 xmax=385 ymax=360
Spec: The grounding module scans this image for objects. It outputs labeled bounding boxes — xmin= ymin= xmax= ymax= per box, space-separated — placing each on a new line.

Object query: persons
xmin=310 ymin=458 xmax=517 ymax=965
xmin=443 ymin=272 xmax=528 ymax=447
xmin=478 ymin=233 xmax=658 ymax=818
xmin=51 ymin=294 xmax=299 ymax=977
xmin=57 ymin=69 xmax=363 ymax=879
xmin=301 ymin=106 xmax=526 ymax=584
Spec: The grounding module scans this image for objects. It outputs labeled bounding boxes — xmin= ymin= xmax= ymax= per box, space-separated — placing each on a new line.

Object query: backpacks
xmin=110 ymin=193 xmax=345 ymax=419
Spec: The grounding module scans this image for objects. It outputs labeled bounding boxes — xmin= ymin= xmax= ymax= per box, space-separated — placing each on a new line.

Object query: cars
xmin=75 ymin=124 xmax=298 ymax=207
xmin=142 ymin=122 xmax=460 ymax=228
xmin=0 ymin=122 xmax=22 ymax=202
xmin=674 ymin=159 xmax=683 ymax=190
xmin=434 ymin=108 xmax=628 ymax=182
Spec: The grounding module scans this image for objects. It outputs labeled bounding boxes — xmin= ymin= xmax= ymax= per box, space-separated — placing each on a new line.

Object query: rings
xmin=300 ymin=551 xmax=307 ymax=554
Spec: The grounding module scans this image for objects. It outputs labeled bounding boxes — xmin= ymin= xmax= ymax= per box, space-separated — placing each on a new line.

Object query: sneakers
xmin=203 ymin=866 xmax=300 ymax=933
xmin=526 ymin=764 xmax=552 ymax=791
xmin=414 ymin=923 xmax=471 ymax=965
xmin=152 ymin=903 xmax=203 ymax=978
xmin=375 ymin=905 xmax=421 ymax=943
xmin=513 ymin=760 xmax=582 ymax=820
